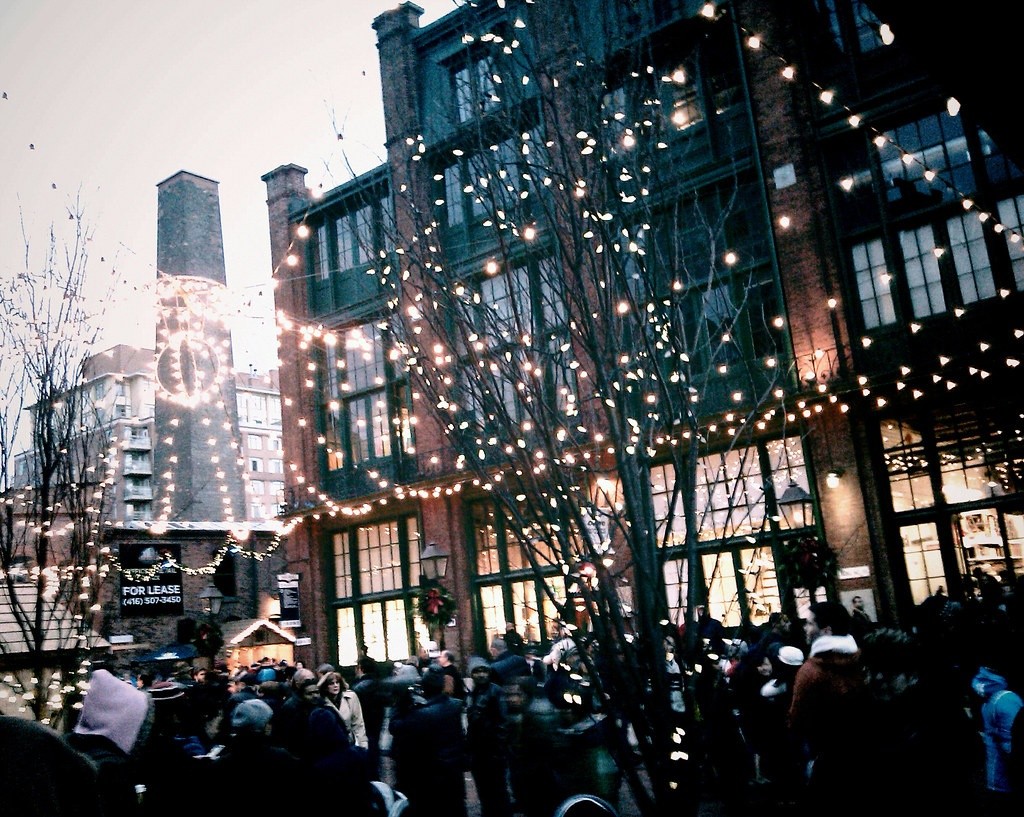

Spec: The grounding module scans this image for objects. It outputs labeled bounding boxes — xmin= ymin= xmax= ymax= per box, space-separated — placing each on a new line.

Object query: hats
xmin=778 ymin=646 xmax=804 ymax=665
xmin=232 ymin=699 xmax=273 ymax=732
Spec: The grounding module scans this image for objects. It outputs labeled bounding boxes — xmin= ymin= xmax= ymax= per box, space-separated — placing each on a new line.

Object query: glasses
xmin=305 ymin=679 xmax=316 ymax=684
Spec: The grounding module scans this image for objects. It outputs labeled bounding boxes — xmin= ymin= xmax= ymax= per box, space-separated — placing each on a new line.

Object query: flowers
xmin=408 ymin=581 xmax=457 ymax=634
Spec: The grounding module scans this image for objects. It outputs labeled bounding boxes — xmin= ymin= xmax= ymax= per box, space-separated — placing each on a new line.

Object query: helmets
xmin=258 ymin=669 xmax=275 ymax=682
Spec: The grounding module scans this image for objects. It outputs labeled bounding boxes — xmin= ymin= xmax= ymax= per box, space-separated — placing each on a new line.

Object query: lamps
xmin=806 ymin=373 xmax=817 ymax=387
xmin=824 ymin=467 xmax=844 ymax=489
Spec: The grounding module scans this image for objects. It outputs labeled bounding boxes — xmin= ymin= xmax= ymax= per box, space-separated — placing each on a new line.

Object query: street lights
xmin=777 ymin=476 xmax=819 ymax=608
xmin=418 ymin=542 xmax=450 ymax=652
xmin=197 ymin=581 xmax=224 ymax=671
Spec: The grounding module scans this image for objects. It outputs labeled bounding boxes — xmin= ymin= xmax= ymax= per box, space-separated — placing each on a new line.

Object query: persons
xmin=0 ymin=568 xmax=1024 ymax=817
